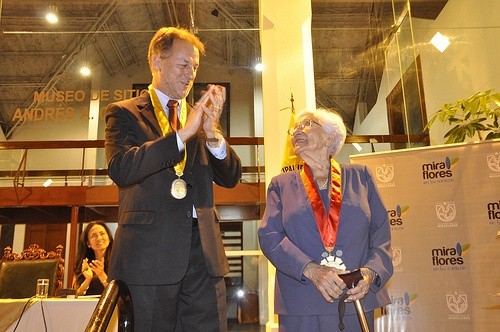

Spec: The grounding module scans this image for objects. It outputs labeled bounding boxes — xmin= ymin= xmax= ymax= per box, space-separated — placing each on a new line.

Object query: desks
xmin=0 ymin=296 xmax=119 ymax=332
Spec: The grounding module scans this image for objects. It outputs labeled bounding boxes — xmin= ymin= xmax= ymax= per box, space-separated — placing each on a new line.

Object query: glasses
xmin=287 ymin=119 xmax=321 ymax=136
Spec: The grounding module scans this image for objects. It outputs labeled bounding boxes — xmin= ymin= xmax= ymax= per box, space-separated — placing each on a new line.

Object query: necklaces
xmin=319 ymin=178 xmax=328 ymax=189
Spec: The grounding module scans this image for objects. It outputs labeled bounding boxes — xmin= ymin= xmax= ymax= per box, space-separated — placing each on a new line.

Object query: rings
xmin=213 ymin=105 xmax=221 ymax=112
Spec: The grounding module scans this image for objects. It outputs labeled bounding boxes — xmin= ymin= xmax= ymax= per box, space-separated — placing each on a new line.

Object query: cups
xmin=36 ymin=279 xmax=49 ymax=299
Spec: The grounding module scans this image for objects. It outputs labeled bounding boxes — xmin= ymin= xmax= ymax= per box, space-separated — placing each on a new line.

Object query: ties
xmin=166 ymin=100 xmax=183 ymax=132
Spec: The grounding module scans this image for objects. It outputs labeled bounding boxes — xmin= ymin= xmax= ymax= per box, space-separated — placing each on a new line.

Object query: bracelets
xmin=101 ymin=275 xmax=108 ymax=283
xmin=204 ymin=128 xmax=222 ymax=142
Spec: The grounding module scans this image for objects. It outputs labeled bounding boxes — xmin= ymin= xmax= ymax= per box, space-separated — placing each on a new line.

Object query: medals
xmin=320 ymin=250 xmax=347 ymax=273
xmin=170 ymin=178 xmax=188 ymax=200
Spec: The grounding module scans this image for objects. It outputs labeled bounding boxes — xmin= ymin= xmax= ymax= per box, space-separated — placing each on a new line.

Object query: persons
xmin=104 ymin=27 xmax=242 ymax=332
xmin=258 ymin=108 xmax=394 ymax=332
xmin=72 ymin=222 xmax=114 ymax=296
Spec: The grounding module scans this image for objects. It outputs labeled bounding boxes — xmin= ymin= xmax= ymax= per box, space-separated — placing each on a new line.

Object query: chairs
xmin=0 ymin=258 xmax=59 ymax=297
